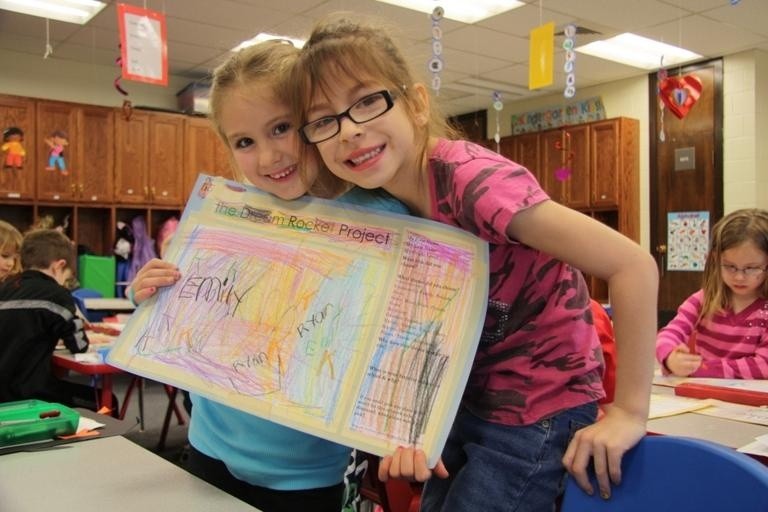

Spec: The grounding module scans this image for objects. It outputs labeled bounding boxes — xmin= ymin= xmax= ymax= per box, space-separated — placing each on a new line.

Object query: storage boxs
xmin=176 ymin=80 xmax=212 ymax=114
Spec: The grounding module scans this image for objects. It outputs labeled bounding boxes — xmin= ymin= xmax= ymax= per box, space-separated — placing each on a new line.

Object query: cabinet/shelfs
xmin=184 ymin=114 xmax=234 ymax=210
xmin=0 ymin=93 xmax=35 ymax=200
xmin=488 ymin=118 xmax=639 ymax=210
xmin=36 ymin=96 xmax=114 ymax=203
xmin=114 ymin=106 xmax=184 ymax=207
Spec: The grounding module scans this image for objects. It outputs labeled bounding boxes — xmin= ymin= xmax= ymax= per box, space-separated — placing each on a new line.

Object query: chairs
xmin=72 ymin=288 xmax=109 ymax=322
xmin=559 ymin=435 xmax=768 ymax=512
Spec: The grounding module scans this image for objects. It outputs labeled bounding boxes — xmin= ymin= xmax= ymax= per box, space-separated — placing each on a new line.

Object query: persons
xmin=0 ymin=220 xmax=25 ymax=284
xmin=1 ymin=230 xmax=122 ymax=419
xmin=122 ymin=37 xmax=452 ymax=511
xmin=655 ymin=208 xmax=768 ymax=381
xmin=290 ymin=14 xmax=662 ymax=512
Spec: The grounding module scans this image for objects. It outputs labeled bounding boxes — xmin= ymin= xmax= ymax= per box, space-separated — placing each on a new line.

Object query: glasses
xmin=718 ymin=259 xmax=768 ymax=277
xmin=296 ymin=84 xmax=403 ymax=149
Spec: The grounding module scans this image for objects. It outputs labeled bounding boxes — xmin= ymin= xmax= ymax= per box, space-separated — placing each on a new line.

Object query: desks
xmin=0 ymin=435 xmax=265 ymax=512
xmin=646 ymin=367 xmax=768 ymax=466
xmin=53 ymin=299 xmax=185 ymax=447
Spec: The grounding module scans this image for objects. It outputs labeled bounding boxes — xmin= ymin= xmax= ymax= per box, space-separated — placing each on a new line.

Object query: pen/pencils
xmin=0 ymin=417 xmax=44 ymax=425
